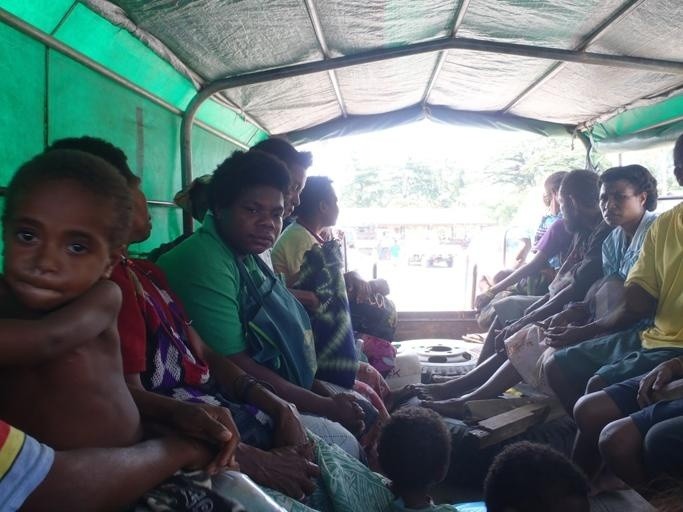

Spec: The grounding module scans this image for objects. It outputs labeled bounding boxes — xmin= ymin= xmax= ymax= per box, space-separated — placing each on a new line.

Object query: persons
xmin=150 ymin=148 xmax=382 ymax=471
xmin=544 ymin=134 xmax=683 ymax=473
xmin=2 ymin=149 xmax=146 ymax=447
xmin=644 ymin=415 xmax=683 ymax=482
xmin=410 ymin=167 xmax=616 ymax=419
xmin=247 ymin=138 xmax=313 ymax=222
xmin=376 ymin=408 xmax=459 ymax=512
xmin=474 ymin=169 xmax=569 ymax=368
xmin=504 ymin=165 xmax=660 ymax=398
xmin=0 ymin=385 xmax=245 ymax=512
xmin=483 ymin=442 xmax=592 ymax=512
xmin=343 ymin=358 xmax=415 ymax=416
xmin=572 ymin=356 xmax=683 ymax=491
xmin=42 ymin=139 xmax=400 ymax=512
xmin=269 ymin=176 xmax=398 ymax=377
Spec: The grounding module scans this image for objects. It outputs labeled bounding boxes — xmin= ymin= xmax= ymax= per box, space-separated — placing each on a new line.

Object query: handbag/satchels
xmin=246 ymin=277 xmax=317 ymax=390
xmin=349 ymin=292 xmax=396 ymax=342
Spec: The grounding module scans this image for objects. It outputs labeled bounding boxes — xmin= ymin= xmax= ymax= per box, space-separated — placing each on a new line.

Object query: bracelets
xmin=675 ymin=355 xmax=683 ymax=371
xmin=485 ymin=290 xmax=497 ymax=300
xmin=231 ymin=373 xmax=258 ymax=402
